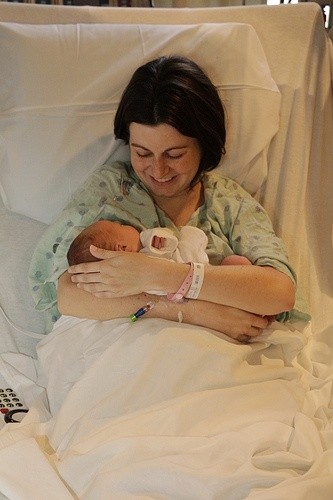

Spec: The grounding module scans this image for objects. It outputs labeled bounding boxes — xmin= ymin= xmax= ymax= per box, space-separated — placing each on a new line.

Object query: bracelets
xmin=185 ymin=262 xmax=204 ymax=300
xmin=168 ymin=262 xmax=194 ymax=303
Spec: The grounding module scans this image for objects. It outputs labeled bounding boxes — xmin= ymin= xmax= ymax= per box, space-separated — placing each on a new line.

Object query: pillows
xmin=1 ymin=21 xmax=281 ymax=226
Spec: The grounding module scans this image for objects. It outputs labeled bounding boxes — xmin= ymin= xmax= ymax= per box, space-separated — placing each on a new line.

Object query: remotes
xmin=0 ymin=388 xmax=29 ymax=424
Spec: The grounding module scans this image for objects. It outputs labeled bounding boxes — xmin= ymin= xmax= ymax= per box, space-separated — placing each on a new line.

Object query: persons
xmin=66 ymin=220 xmax=278 ymax=327
xmin=29 ymin=55 xmax=297 ymax=342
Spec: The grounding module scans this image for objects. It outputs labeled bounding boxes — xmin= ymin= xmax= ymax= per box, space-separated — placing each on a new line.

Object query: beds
xmin=0 ymin=3 xmax=333 ymax=500
